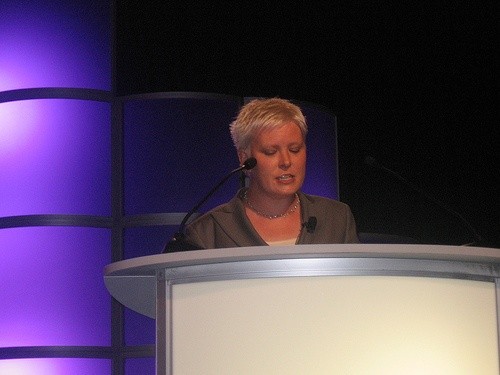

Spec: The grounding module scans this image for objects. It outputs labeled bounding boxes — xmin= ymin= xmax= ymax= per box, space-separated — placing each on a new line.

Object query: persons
xmin=176 ymin=98 xmax=358 ymax=249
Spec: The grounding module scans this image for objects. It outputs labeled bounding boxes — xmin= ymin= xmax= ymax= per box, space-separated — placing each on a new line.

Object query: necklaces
xmin=242 ymin=190 xmax=300 ymax=219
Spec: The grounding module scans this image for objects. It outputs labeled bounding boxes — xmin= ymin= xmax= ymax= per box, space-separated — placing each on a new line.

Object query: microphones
xmin=364 ymin=157 xmax=497 ymax=249
xmin=162 ymin=157 xmax=258 ymax=254
xmin=306 ymin=216 xmax=317 ymax=233
